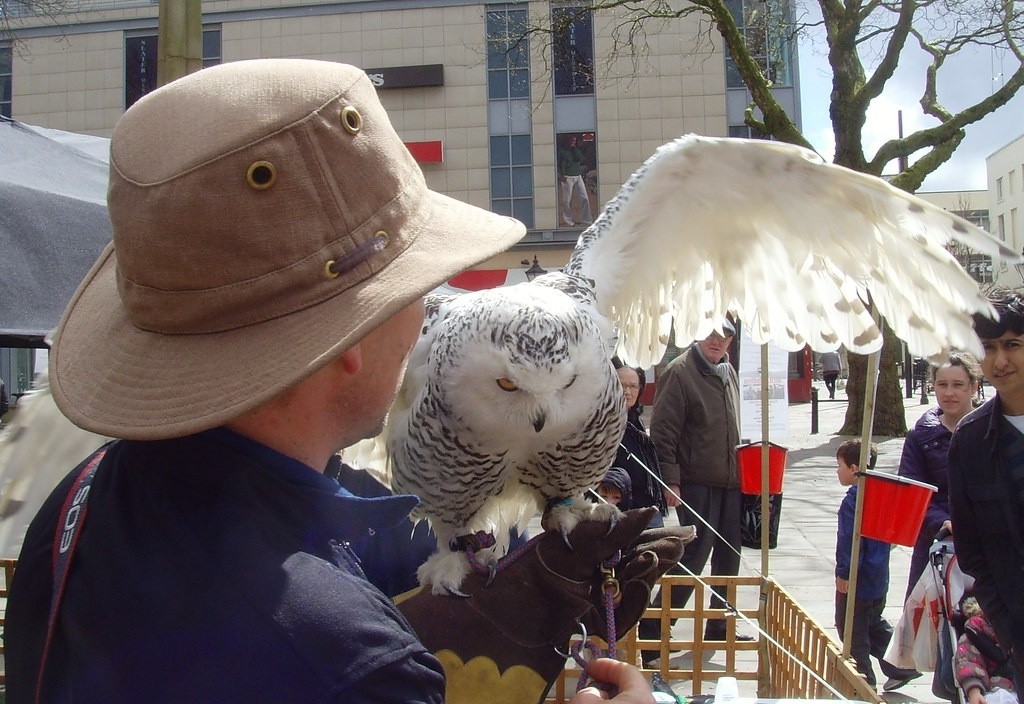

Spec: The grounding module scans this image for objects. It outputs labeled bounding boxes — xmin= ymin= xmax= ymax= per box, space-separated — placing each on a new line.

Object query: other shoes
xmin=704 ymin=631 xmax=755 ymax=642
xmin=669 ymin=636 xmax=680 ymax=652
xmin=883 ymin=672 xmax=922 ymax=690
xmin=643 ymin=659 xmax=679 ymax=669
xmin=870 ymin=685 xmax=877 ymax=694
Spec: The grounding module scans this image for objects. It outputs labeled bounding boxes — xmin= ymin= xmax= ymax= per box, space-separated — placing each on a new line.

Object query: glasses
xmin=621 ymin=382 xmax=641 ymax=390
xmin=706 ymin=333 xmax=733 ymax=341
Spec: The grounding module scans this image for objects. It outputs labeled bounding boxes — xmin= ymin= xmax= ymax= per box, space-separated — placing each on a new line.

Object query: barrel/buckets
xmin=861 ymin=469 xmax=938 ymax=547
xmin=735 ymin=442 xmax=785 ymax=495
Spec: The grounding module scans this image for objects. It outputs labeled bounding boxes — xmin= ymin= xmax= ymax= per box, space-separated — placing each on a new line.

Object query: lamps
xmin=524 ymin=254 xmax=546 ymax=282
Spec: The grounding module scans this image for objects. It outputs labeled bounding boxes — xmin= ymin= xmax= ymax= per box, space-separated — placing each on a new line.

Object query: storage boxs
xmin=735 ymin=443 xmax=788 ymax=494
xmin=861 ymin=468 xmax=939 ymax=547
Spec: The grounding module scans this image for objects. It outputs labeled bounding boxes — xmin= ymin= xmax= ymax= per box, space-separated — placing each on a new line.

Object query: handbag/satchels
xmin=741 ymin=493 xmax=783 ymax=549
xmin=913 ymin=566 xmax=946 ymax=672
xmin=883 ymin=551 xmax=932 ymax=669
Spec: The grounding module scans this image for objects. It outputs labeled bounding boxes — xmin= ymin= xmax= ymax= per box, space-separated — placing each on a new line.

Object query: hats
xmin=47 ymin=58 xmax=527 ymax=438
xmin=723 ymin=318 xmax=735 ymax=335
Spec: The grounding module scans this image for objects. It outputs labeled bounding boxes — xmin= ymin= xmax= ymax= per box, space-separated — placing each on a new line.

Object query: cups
xmin=713 ymin=677 xmax=738 ymax=702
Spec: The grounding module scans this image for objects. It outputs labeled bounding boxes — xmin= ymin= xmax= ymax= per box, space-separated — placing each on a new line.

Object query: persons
xmin=557 ymin=136 xmax=592 ymax=228
xmin=650 ymin=310 xmax=755 ymax=653
xmin=836 ymin=440 xmax=924 ymax=692
xmin=883 ymin=353 xmax=985 ymax=691
xmin=818 ymin=351 xmax=842 ymax=398
xmin=588 ymin=467 xmax=632 ymax=513
xmin=947 ymin=284 xmax=1023 ymax=704
xmin=954 ymin=596 xmax=1019 ymax=703
xmin=609 ymin=355 xmax=679 ymax=673
xmin=5 ymin=58 xmax=695 ymax=704
